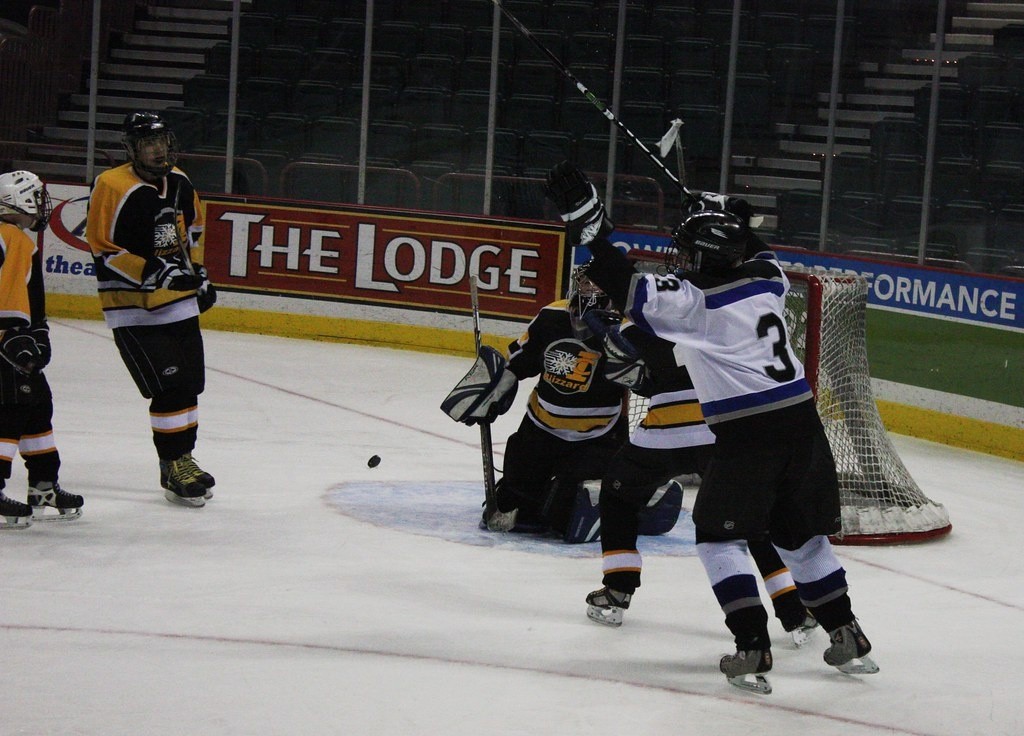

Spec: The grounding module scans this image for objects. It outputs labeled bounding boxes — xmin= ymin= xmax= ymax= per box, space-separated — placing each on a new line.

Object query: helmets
xmin=122 ymin=112 xmax=179 ymax=177
xmin=665 ymin=210 xmax=747 ymax=290
xmin=573 ymin=260 xmax=609 ymax=313
xmin=0 ymin=171 xmax=53 ymax=231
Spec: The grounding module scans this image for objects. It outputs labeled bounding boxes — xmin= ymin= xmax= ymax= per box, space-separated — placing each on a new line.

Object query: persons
xmin=546 ymin=138 xmax=872 ymax=677
xmin=0 ymin=170 xmax=84 ymax=517
xmin=483 ymin=264 xmax=645 ymax=529
xmin=84 ymin=110 xmax=216 ymax=497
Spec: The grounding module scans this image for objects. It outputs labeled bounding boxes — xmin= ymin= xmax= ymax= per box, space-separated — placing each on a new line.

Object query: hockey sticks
xmin=491 ymin=0 xmax=716 ymax=210
xmin=0 ymin=351 xmax=37 ymax=378
xmin=469 ymin=274 xmax=520 ymax=532
xmin=173 ymin=177 xmax=197 ymax=276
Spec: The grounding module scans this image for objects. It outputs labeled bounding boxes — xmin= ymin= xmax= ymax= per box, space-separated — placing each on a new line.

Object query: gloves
xmin=141 ymin=256 xmax=203 ymax=292
xmin=29 ymin=322 xmax=51 ymax=368
xmin=680 ymin=192 xmax=751 ymax=228
xmin=542 ymin=161 xmax=615 ymax=246
xmin=191 ymin=263 xmax=217 ymax=314
xmin=1 ymin=327 xmax=41 ymax=373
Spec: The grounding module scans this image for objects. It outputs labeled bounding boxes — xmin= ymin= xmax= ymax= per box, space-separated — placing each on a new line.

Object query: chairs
xmin=0 ymin=0 xmax=1024 ymax=276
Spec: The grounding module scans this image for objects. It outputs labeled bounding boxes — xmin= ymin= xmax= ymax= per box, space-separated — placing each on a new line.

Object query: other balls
xmin=366 ymin=454 xmax=381 ymax=468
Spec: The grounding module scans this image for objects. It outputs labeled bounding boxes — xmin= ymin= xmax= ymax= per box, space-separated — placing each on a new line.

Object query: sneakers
xmin=781 ymin=606 xmax=821 ymax=648
xmin=585 ymin=586 xmax=634 ymax=627
xmin=27 ymin=481 xmax=84 ymax=522
xmin=823 ymin=619 xmax=880 ymax=674
xmin=719 ymin=648 xmax=773 ymax=694
xmin=181 ymin=451 xmax=215 ymax=499
xmin=159 ymin=456 xmax=206 ymax=506
xmin=0 ymin=491 xmax=33 ymax=530
